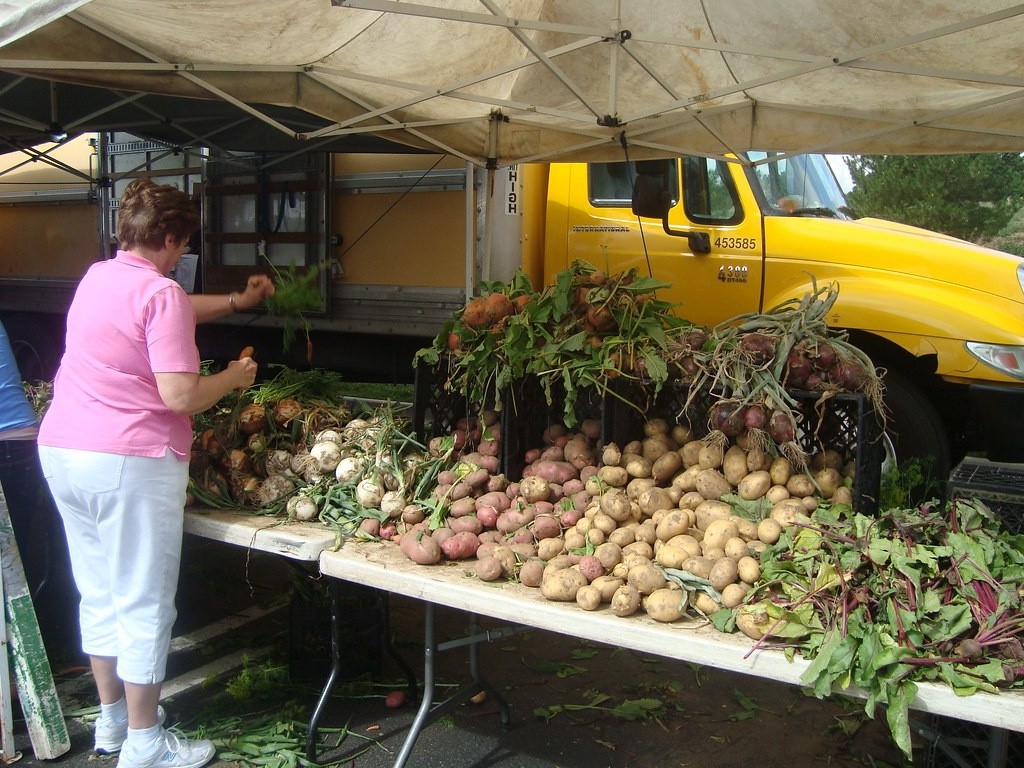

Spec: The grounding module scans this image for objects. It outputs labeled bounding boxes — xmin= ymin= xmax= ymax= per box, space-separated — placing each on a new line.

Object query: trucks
xmin=0 ymin=131 xmax=1024 ymax=500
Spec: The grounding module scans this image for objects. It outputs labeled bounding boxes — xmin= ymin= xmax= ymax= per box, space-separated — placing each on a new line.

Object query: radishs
xmin=183 ymin=396 xmax=443 ymax=523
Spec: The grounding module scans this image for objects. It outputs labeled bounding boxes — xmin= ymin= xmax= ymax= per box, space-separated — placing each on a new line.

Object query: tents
xmin=1 ymin=2 xmax=1024 ymax=189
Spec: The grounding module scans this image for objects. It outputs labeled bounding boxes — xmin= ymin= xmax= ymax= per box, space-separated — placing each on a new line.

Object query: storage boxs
xmin=412 ymin=355 xmax=601 ymax=481
xmin=613 ymin=374 xmax=881 ymax=519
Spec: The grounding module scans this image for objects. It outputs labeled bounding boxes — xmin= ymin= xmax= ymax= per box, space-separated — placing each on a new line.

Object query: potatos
xmin=386 ymin=692 xmax=404 ymax=707
xmin=362 ymin=410 xmax=855 ymax=639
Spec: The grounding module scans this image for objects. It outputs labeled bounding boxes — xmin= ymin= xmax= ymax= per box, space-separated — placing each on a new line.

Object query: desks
xmin=182 ymin=505 xmax=418 ymax=761
xmin=319 ymin=524 xmax=1024 ymax=767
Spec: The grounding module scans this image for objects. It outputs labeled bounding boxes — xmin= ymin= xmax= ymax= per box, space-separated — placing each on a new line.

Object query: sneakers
xmin=115 ymin=721 xmax=216 ymax=768
xmin=93 ymin=705 xmax=167 ymax=756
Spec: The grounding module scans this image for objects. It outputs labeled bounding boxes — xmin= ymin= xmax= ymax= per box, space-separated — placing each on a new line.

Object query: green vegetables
xmin=169 ymin=656 xmax=367 ymax=768
xmin=532 ymin=648 xmax=757 ymax=722
xmin=711 ymin=460 xmax=1024 ymax=762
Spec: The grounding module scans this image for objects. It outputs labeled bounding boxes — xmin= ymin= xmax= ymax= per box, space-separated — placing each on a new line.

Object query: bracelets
xmin=229 ymin=291 xmax=238 ymax=308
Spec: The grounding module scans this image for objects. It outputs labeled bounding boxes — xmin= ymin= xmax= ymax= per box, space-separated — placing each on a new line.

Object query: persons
xmin=0 ymin=322 xmax=92 ymax=733
xmin=36 ymin=179 xmax=275 ymax=768
